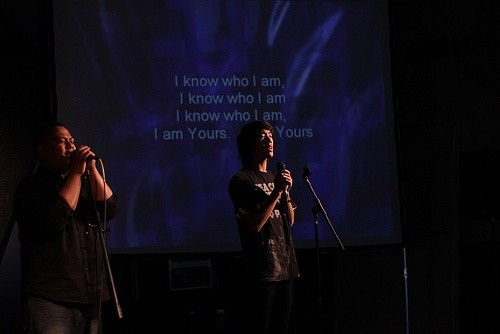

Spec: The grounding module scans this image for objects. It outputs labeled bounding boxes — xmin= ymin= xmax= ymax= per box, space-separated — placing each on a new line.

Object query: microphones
xmin=85 ymin=154 xmax=99 ymax=160
xmin=276 ymin=161 xmax=285 ymax=171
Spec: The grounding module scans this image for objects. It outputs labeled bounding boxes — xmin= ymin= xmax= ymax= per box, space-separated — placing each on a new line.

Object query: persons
xmin=228 ymin=120 xmax=300 ymax=334
xmin=13 ymin=121 xmax=119 ymax=334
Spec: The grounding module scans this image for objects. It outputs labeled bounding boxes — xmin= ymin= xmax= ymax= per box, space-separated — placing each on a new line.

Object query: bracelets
xmin=288 ymin=200 xmax=292 ymax=203
